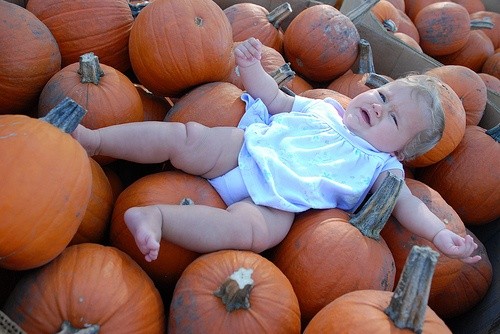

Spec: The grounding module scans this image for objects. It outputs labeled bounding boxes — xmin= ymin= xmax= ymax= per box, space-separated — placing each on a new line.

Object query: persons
xmin=70 ymin=36 xmax=482 ymax=264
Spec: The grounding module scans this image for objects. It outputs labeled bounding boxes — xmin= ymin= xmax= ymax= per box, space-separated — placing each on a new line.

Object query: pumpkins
xmin=0 ymin=0 xmax=500 ymax=334
